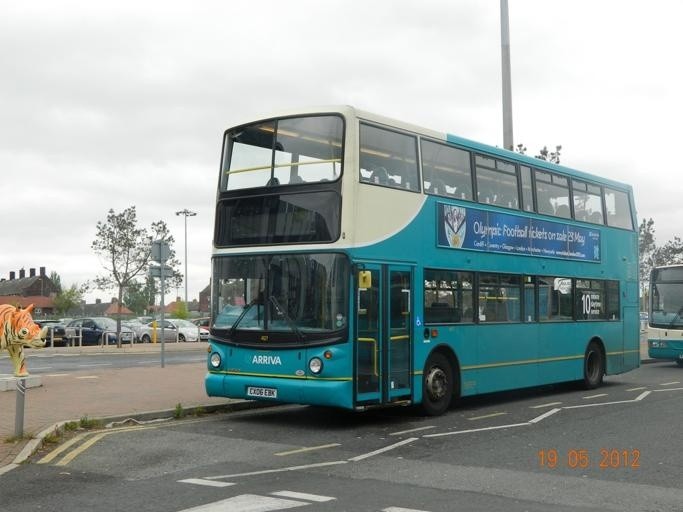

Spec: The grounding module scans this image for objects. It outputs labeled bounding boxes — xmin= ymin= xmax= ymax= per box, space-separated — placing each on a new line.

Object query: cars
xmin=23 ymin=314 xmax=211 ymax=350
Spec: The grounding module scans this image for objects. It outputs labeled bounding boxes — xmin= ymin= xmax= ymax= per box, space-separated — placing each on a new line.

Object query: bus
xmin=202 ymin=101 xmax=645 ymax=421
xmin=647 ymin=261 xmax=683 ymax=367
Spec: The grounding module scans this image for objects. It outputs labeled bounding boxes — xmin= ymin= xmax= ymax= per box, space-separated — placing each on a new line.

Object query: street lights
xmin=37 ymin=277 xmax=43 ymax=308
xmin=175 ymin=209 xmax=199 ymax=313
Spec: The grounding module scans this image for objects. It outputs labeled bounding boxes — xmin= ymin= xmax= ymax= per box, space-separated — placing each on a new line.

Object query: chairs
xmin=365 ymin=166 xmax=602 ymax=225
